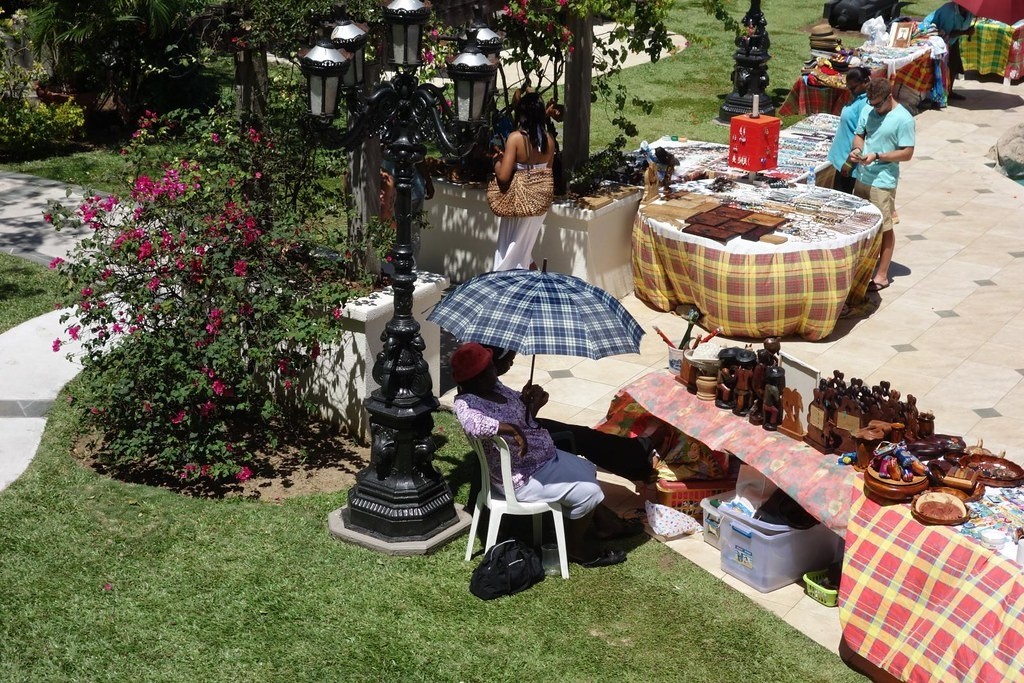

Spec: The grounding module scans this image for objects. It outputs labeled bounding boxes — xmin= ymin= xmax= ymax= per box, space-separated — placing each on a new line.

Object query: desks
xmin=623 ymin=356 xmax=1024 ymax=683
xmin=803 ymin=37 xmax=948 ymax=114
xmin=629 ymin=113 xmax=885 ymax=344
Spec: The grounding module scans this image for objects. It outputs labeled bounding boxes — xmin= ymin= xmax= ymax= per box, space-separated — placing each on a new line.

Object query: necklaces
xmin=657 ymin=114 xmax=881 ymax=242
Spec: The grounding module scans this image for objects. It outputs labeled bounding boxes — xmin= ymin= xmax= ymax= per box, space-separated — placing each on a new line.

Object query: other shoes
xmin=647 ymin=425 xmax=668 ymax=446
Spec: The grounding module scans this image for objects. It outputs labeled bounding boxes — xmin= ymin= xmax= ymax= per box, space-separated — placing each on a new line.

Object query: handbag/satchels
xmin=468 ymin=535 xmax=545 ymax=600
xmin=487 ymin=128 xmax=554 ymax=217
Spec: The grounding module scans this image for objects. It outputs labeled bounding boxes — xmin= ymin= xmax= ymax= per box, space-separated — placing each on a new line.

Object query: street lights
xmin=295 ymin=0 xmax=503 ymax=555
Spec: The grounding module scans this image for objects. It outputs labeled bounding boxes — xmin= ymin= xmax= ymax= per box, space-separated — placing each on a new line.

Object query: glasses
xmin=866 ymin=92 xmax=891 ymax=107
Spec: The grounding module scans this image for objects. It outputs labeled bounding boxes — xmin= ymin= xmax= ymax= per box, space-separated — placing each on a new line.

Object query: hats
xmin=450 ymin=342 xmax=493 ymax=382
xmin=809 ymin=23 xmax=838 ymax=40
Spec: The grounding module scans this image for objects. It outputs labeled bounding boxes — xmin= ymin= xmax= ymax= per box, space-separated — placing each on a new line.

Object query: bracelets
xmin=875 ymin=152 xmax=879 ymax=159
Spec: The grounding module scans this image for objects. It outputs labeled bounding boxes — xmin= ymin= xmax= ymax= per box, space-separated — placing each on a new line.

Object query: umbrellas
xmin=952 ymin=0 xmax=1024 ymax=43
xmin=424 ymin=256 xmax=646 ymax=430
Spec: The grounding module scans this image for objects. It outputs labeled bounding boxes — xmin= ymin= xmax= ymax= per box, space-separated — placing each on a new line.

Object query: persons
xmin=922 ymin=1 xmax=976 ymax=103
xmin=446 ymin=342 xmax=644 ymax=567
xmin=342 ymin=134 xmax=437 ymax=260
xmin=847 ymin=77 xmax=916 ymax=292
xmin=479 ymin=339 xmax=678 ymax=486
xmin=713 ymin=346 xmax=787 ymax=433
xmin=824 ymin=66 xmax=876 ymax=193
xmin=484 ymin=91 xmax=557 ymax=272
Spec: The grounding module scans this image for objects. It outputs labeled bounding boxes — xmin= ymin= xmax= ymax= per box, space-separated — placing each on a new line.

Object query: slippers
xmin=867 ymin=280 xmax=889 ymax=291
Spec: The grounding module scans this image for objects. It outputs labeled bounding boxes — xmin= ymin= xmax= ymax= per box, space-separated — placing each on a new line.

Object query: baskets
xmin=654 ymin=484 xmax=738 ymax=518
xmin=802 ymin=568 xmax=838 ymax=607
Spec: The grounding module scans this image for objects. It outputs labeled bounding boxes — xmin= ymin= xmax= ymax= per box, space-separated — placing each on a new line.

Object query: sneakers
xmin=583 ymin=549 xmax=627 ymax=566
xmin=948 ymin=91 xmax=966 ymax=100
xmin=602 ymin=521 xmax=644 ymax=539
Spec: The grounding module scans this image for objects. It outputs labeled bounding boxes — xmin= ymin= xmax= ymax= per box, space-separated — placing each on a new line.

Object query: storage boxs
xmin=655 ymin=472 xmax=841 ymax=609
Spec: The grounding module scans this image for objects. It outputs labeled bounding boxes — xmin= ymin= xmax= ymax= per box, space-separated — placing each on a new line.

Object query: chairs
xmin=462 ymin=427 xmax=570 ymax=579
